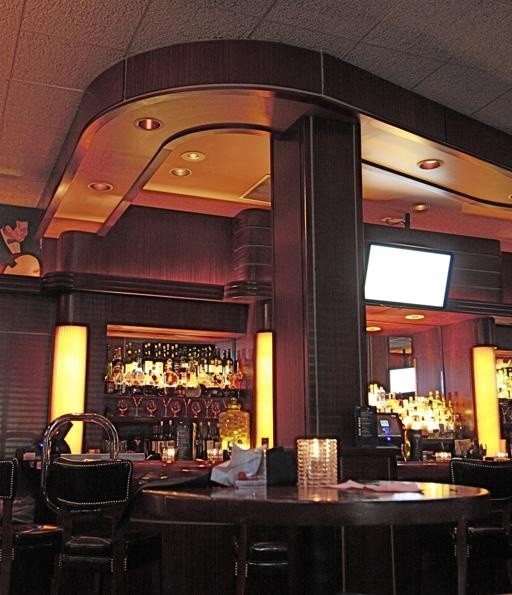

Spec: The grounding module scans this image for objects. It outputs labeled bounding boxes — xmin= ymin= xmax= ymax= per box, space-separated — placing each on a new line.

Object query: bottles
xmin=130 ymin=420 xmax=222 ymax=462
xmin=217 ymin=397 xmax=250 ymax=453
xmin=106 ymin=342 xmax=237 ymax=396
xmin=369 ymin=381 xmax=457 ymax=434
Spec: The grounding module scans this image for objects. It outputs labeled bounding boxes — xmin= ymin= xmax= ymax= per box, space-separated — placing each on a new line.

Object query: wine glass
xmin=115 ymin=395 xmax=222 ymax=420
xmin=480 ymin=443 xmax=487 ymax=460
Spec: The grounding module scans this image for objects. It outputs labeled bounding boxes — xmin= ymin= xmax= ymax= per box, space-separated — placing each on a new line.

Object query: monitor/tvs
xmin=377 ymin=413 xmax=403 ymax=446
xmin=363 ymin=241 xmax=454 ymax=311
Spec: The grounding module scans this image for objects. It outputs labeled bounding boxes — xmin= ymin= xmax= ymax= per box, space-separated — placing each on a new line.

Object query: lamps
xmin=472 ymin=345 xmax=502 ymax=457
xmin=47 ymin=324 xmax=89 ymax=454
xmin=254 ymin=330 xmax=275 ymax=450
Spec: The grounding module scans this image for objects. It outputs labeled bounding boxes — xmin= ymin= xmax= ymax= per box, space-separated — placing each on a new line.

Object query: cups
xmin=296 ymin=437 xmax=339 ymax=489
xmin=236 ymin=446 xmax=268 ymax=487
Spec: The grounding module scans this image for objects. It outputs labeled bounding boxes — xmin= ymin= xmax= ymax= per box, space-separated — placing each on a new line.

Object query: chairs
xmin=0 ymin=454 xmax=132 ymax=593
xmin=339 ymin=439 xmax=403 ymax=496
xmin=446 ymin=457 xmax=512 ymax=564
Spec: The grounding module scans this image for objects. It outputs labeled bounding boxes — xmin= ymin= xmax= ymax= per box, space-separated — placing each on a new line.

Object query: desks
xmin=139 ymin=470 xmax=490 ymax=595
